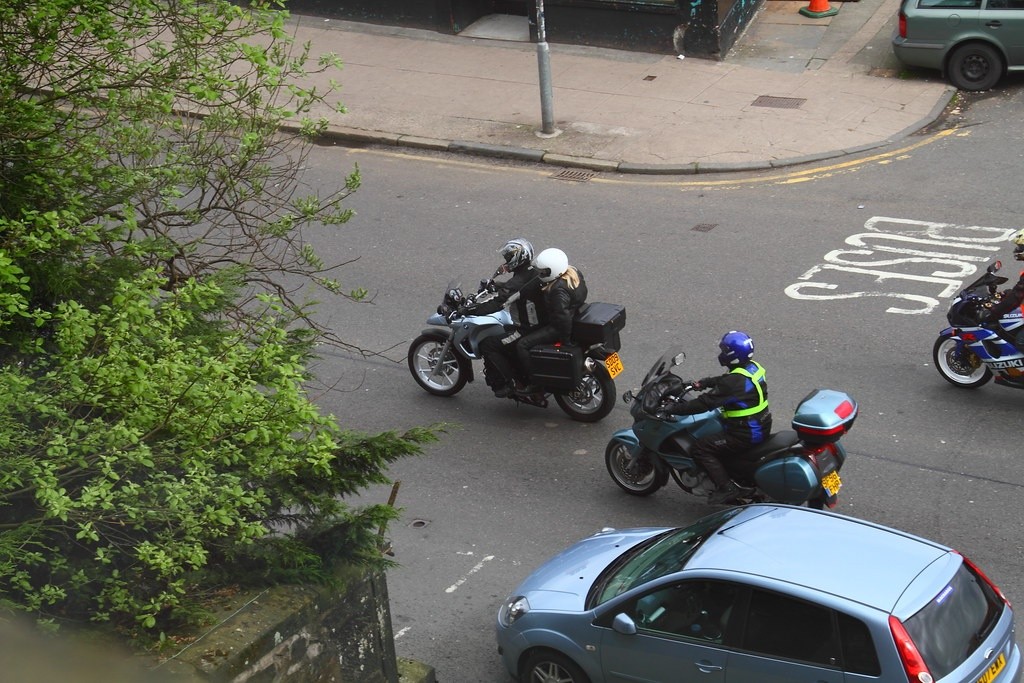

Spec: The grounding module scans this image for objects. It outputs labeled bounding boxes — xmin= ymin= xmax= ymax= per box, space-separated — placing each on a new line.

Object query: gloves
xmin=977 ymin=310 xmax=993 ymax=323
xmin=494 ymin=282 xmax=505 ymax=291
xmin=457 ymin=306 xmax=469 ymax=318
xmin=1000 ymin=289 xmax=1012 ymax=302
xmin=664 ymin=401 xmax=673 ymax=413
xmin=698 ymin=378 xmax=708 ymax=390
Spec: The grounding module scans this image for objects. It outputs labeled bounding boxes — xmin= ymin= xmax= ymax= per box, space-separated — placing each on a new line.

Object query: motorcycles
xmin=933 ymin=261 xmax=1024 ymax=389
xmin=408 ymin=263 xmax=626 ymax=422
xmin=605 ymin=345 xmax=859 ymax=510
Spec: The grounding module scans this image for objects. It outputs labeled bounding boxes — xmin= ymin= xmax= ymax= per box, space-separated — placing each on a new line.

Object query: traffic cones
xmin=798 ymin=0 xmax=839 ymax=19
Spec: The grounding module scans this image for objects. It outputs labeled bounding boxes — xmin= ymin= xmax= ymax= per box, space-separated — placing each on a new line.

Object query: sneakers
xmin=708 ymin=481 xmax=743 ymax=506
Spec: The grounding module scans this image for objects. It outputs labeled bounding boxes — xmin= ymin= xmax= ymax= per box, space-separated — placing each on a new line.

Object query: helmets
xmin=534 ymin=248 xmax=568 ymax=283
xmin=497 ymin=237 xmax=534 ymax=273
xmin=718 ymin=330 xmax=755 ymax=366
xmin=1011 ymin=227 xmax=1024 ymax=261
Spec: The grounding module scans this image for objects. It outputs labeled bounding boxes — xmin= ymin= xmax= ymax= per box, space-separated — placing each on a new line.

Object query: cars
xmin=891 ymin=0 xmax=1024 ymax=92
xmin=495 ymin=503 xmax=1020 ymax=683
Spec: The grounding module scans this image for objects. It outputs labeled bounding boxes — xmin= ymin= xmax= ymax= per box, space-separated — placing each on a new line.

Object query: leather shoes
xmin=512 ymin=382 xmax=544 ymax=396
xmin=495 ymin=375 xmax=523 ymax=398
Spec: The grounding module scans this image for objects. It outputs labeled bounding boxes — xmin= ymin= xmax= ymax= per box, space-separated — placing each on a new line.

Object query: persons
xmin=978 ymin=228 xmax=1024 ymax=352
xmin=513 ymin=249 xmax=588 ymax=398
xmin=656 ymin=331 xmax=773 ymax=507
xmin=458 ymin=238 xmax=548 ymax=399
